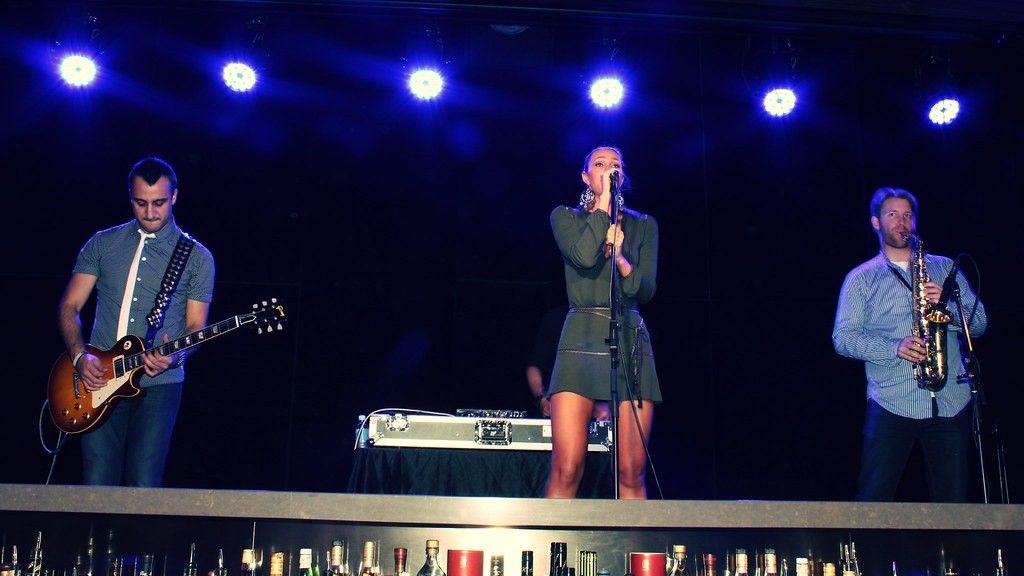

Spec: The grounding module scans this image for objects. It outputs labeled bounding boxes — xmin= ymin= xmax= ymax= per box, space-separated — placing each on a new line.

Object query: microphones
xmin=610 ymin=170 xmax=619 ymax=180
xmin=937 ymin=256 xmax=961 ymax=309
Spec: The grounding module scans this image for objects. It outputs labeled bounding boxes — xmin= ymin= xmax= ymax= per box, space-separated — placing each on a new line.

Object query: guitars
xmin=46 ymin=295 xmax=289 ymax=437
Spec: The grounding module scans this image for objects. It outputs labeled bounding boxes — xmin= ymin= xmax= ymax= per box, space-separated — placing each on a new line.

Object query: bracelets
xmin=169 ymin=351 xmax=186 ymax=370
xmin=73 ymin=351 xmax=88 ymax=367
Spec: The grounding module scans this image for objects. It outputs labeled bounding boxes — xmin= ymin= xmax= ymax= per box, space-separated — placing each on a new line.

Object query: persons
xmin=60 ymin=157 xmax=215 ymax=487
xmin=832 ymin=188 xmax=987 ymax=502
xmin=527 ymin=301 xmax=610 ymax=420
xmin=545 ymin=147 xmax=663 ymax=500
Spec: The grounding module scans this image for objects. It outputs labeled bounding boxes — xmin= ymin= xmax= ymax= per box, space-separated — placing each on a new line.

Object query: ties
xmin=116 ymin=229 xmax=156 ymax=341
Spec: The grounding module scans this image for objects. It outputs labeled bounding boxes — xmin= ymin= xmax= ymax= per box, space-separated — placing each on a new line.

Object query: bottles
xmin=356 ymin=414 xmax=369 ymax=448
xmin=0 ymin=532 xmax=1006 ymax=576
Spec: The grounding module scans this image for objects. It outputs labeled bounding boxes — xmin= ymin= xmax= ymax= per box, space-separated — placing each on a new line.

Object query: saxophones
xmin=899 ymin=230 xmax=956 ymax=393
